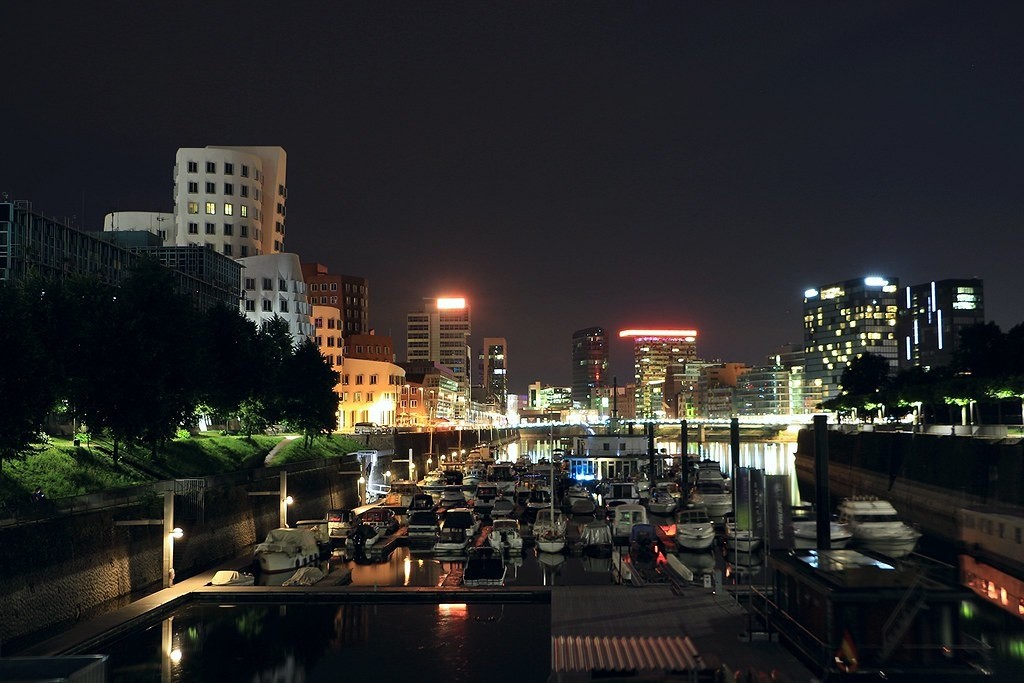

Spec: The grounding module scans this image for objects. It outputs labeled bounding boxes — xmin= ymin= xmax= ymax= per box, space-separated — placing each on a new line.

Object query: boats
xmin=291 ymin=449 xmax=760 ymax=556
xmin=461 ymin=544 xmax=510 ymax=587
xmin=837 ymin=495 xmax=924 ymax=558
xmin=794 ymin=426 xmax=1024 ymax=573
xmin=792 ymin=506 xmax=854 ymax=550
xmin=255 ymin=526 xmax=321 ymax=575
